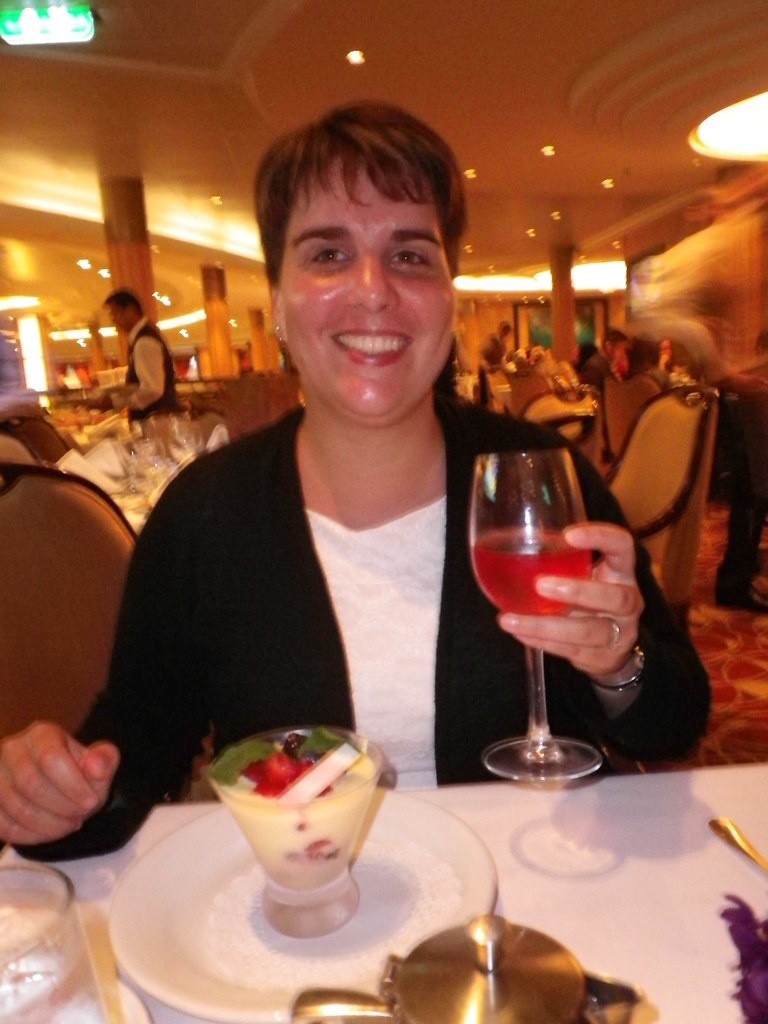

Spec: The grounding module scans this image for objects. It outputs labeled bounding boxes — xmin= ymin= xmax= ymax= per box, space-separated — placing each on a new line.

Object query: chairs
xmin=0 ymin=369 xmax=716 ymax=738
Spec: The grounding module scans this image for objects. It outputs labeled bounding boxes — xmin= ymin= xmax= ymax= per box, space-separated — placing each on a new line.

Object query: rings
xmin=608 ymin=618 xmax=619 ymax=647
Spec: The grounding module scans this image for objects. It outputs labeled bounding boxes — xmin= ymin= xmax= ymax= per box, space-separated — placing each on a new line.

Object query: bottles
xmin=469 ymin=452 xmax=603 ymax=779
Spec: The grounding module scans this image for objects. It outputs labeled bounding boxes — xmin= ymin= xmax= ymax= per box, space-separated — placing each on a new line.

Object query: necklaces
xmin=0 ymin=99 xmax=709 ymax=863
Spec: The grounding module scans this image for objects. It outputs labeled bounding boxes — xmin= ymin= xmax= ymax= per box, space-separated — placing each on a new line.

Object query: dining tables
xmin=0 ymin=758 xmax=766 ymax=1024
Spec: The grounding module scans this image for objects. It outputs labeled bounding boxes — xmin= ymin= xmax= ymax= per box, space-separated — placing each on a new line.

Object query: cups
xmin=209 ymin=727 xmax=387 ymax=944
xmin=1 ymin=861 xmax=104 ymax=1024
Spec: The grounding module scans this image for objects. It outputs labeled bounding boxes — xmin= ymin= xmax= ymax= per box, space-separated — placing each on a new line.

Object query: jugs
xmin=290 ymin=913 xmax=644 ymax=1023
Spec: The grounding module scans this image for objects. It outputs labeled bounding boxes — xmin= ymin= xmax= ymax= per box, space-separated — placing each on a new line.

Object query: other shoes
xmin=716 ymin=583 xmax=768 ymax=611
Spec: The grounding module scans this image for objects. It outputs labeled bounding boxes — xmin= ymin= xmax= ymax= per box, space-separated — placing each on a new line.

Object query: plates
xmin=107 ymin=785 xmax=498 ymax=1024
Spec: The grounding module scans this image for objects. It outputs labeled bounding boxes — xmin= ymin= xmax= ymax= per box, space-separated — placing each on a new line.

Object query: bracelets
xmin=594 ymin=646 xmax=645 ymax=692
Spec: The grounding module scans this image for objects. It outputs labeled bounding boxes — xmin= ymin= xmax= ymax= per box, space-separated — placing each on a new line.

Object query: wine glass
xmin=117 ymin=425 xmax=205 ymax=513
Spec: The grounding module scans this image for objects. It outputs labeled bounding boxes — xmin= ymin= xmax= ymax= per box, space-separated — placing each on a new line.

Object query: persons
xmin=586 ymin=327 xmax=627 ymax=386
xmin=477 ymin=322 xmax=509 ymax=400
xmin=96 ymin=290 xmax=178 ymax=424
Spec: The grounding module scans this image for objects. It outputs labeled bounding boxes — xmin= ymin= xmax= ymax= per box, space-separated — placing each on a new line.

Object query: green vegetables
xmin=213 ymin=726 xmax=347 ymax=788
